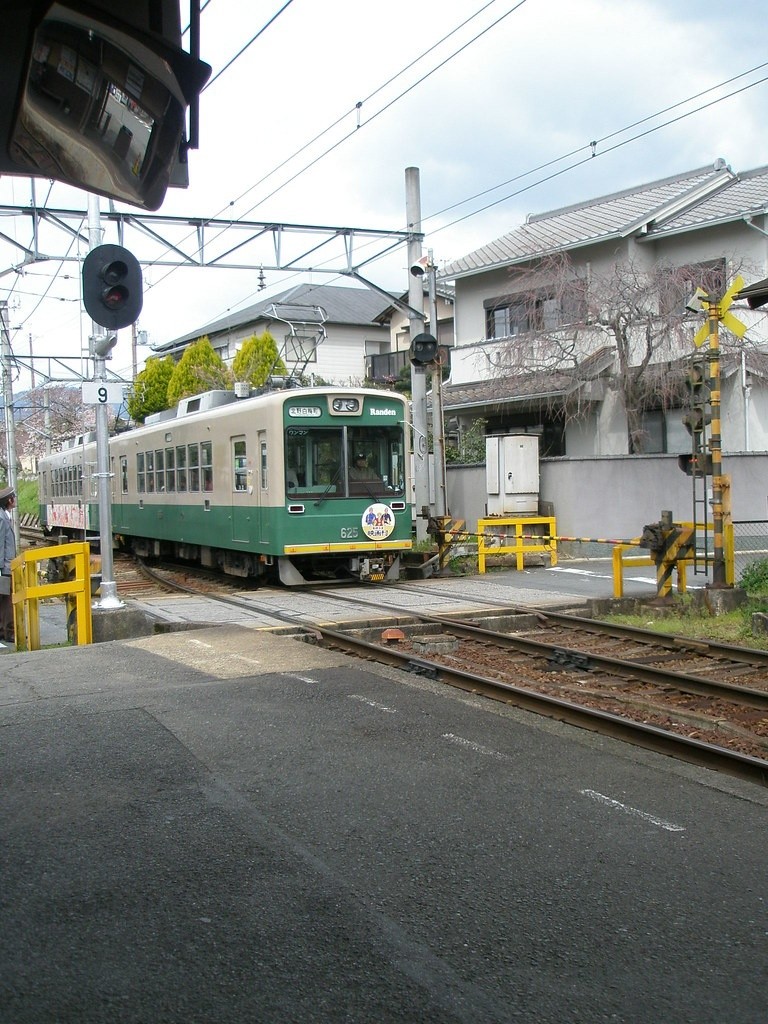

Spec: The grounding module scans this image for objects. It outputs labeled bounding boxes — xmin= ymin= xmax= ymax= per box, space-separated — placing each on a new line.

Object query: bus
xmin=39 ymin=301 xmax=429 ymax=588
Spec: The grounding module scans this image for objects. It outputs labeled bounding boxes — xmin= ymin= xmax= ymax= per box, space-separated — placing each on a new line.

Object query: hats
xmin=0 ymin=487 xmax=15 ymax=503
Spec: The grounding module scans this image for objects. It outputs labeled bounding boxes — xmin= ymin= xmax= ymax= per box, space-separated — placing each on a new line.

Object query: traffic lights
xmin=82 ymin=244 xmax=144 ymax=330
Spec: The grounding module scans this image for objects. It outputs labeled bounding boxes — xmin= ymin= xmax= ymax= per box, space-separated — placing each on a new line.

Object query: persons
xmin=0 ymin=487 xmax=27 ymax=642
xmin=288 ymin=467 xmax=300 ymax=487
xmin=350 ymin=454 xmax=380 ymax=481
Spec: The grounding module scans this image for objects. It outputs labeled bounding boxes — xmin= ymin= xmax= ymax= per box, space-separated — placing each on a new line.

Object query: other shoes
xmin=0 ymin=635 xmax=28 ymax=643
